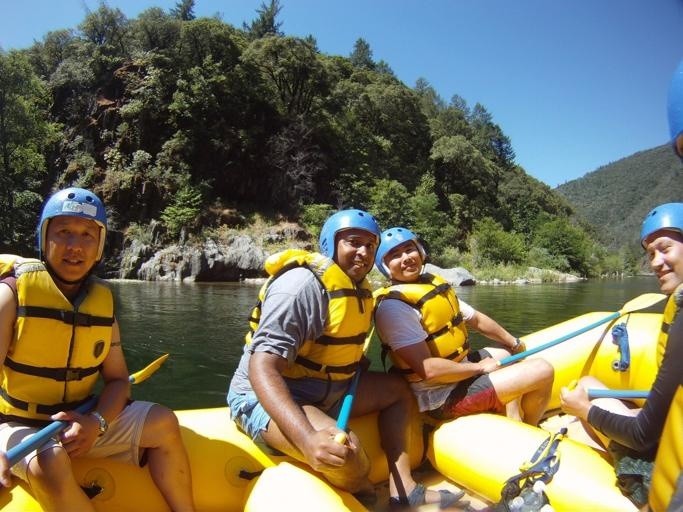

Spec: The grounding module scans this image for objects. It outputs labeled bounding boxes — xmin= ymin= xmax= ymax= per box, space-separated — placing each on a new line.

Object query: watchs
xmin=90 ymin=410 xmax=107 ymax=435
xmin=511 ymin=338 xmax=521 ymax=352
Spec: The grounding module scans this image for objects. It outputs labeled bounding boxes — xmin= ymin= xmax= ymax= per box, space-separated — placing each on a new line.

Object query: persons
xmin=557 ymin=202 xmax=683 ymax=512
xmin=374 ymin=227 xmax=554 ymax=427
xmin=226 ymin=207 xmax=465 ymax=512
xmin=0 ymin=188 xmax=195 ymax=512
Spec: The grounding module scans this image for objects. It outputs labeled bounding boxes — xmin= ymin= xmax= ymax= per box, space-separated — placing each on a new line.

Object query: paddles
xmin=0 ymin=353 xmax=172 ymax=470
xmin=494 ymin=292 xmax=668 ymax=366
xmin=333 ymin=286 xmax=384 ymax=448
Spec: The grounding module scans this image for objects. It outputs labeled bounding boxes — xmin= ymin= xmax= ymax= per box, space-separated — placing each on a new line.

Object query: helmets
xmin=374 ymin=227 xmax=427 ymax=280
xmin=639 ymin=202 xmax=683 ymax=251
xmin=37 ymin=187 xmax=108 ymax=264
xmin=666 ymin=59 xmax=683 ymax=158
xmin=319 ymin=209 xmax=383 ymax=275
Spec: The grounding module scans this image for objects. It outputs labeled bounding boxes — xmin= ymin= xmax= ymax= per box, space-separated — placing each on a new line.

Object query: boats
xmin=1 ymin=311 xmax=681 ymax=512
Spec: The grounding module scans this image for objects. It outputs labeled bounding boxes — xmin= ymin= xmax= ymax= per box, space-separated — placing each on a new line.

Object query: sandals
xmin=387 ymin=483 xmax=466 ymax=512
